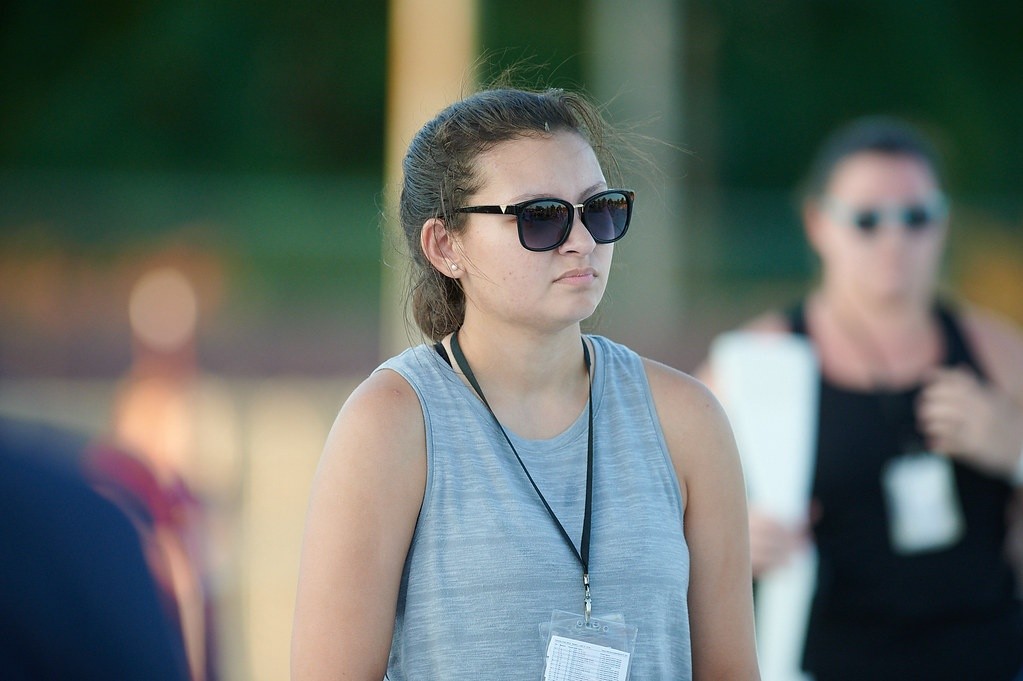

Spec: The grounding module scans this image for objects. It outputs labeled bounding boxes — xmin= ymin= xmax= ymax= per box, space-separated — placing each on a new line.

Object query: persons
xmin=692 ymin=117 xmax=1023 ymax=681
xmin=291 ymin=86 xmax=759 ymax=681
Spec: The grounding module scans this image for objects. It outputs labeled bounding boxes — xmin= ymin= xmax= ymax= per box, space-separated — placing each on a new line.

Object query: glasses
xmin=437 ymin=189 xmax=634 ymax=252
xmin=815 ymin=204 xmax=949 ymax=231
